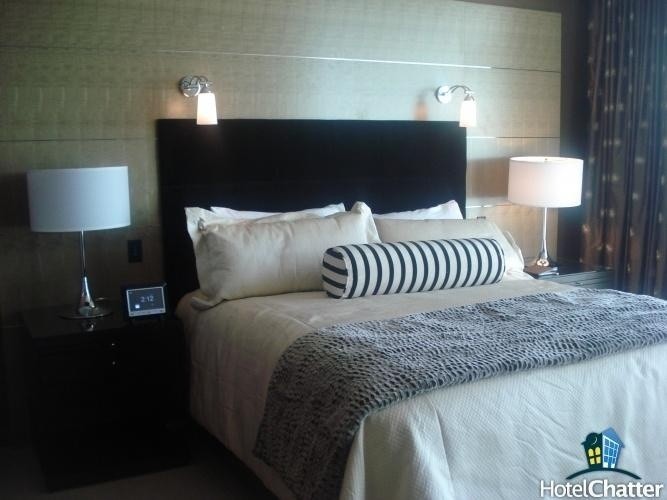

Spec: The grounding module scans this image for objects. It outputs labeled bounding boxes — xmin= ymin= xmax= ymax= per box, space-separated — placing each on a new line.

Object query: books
xmin=523 ymin=265 xmax=559 ymax=277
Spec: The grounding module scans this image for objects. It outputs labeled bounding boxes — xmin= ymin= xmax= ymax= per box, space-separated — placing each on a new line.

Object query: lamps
xmin=434 ymin=83 xmax=480 ymax=126
xmin=175 ymin=68 xmax=221 ymax=129
xmin=506 ymin=155 xmax=585 ymax=280
xmin=18 ymin=166 xmax=134 ymax=320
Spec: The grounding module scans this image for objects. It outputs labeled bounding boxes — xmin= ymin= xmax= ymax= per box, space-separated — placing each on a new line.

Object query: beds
xmin=149 ymin=117 xmax=666 ymax=500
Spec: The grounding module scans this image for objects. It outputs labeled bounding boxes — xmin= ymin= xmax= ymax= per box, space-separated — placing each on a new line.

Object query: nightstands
xmin=16 ymin=301 xmax=196 ymax=490
xmin=519 ymin=256 xmax=614 ymax=297
